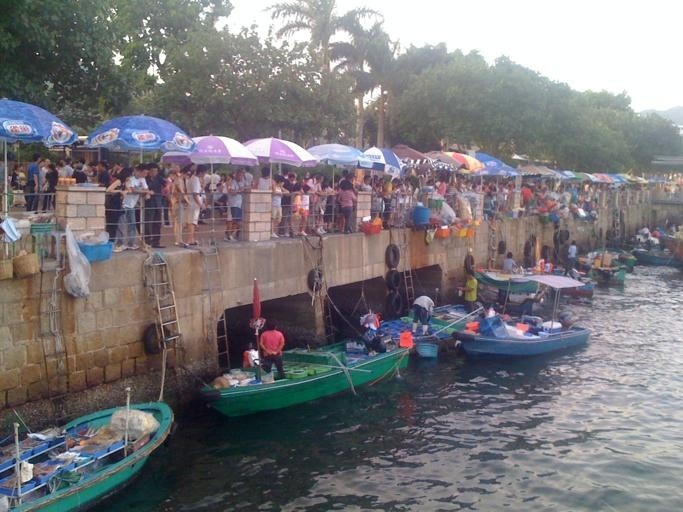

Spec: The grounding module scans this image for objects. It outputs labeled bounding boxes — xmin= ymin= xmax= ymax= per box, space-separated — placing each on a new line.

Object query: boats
xmin=190 ymin=319 xmax=416 ymax=419
xmin=0 ymin=398 xmax=174 ymax=512
xmin=371 ymin=302 xmax=516 ymax=356
xmin=468 ymin=226 xmax=682 ymax=307
xmin=452 ymin=313 xmax=590 ymax=358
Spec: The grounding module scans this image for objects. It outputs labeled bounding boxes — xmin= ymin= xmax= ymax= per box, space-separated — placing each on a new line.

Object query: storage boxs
xmin=0 ymin=435 xmax=152 ymax=497
xmin=541 ymin=321 xmax=563 ymax=332
xmin=272 ymin=361 xmax=332 ymax=379
xmin=78 ymin=240 xmax=112 ymax=263
xmin=381 ymin=319 xmax=449 ymax=339
xmin=361 ymin=220 xmax=380 ymax=234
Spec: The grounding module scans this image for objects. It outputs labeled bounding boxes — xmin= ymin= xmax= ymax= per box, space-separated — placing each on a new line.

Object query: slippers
xmin=187 ymin=240 xmax=200 ymax=245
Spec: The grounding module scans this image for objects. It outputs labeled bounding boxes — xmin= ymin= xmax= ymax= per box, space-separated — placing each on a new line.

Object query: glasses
xmin=187 ymin=174 xmax=191 ymax=178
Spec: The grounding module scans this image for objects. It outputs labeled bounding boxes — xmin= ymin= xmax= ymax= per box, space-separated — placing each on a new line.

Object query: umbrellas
xmin=1 ymin=95 xmax=77 ymax=217
xmin=391 ymin=144 xmax=436 ymax=174
xmin=83 ymin=115 xmax=196 ymax=167
xmin=306 ymin=144 xmax=375 ymax=194
xmin=162 ymin=133 xmax=260 ymax=191
xmin=241 ymin=137 xmax=316 ymax=187
xmin=365 ymin=149 xmax=403 ymax=179
xmin=249 ymin=277 xmax=266 ymax=349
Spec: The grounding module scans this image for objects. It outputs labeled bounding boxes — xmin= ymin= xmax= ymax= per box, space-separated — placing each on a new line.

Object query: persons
xmin=456 ymin=221 xmax=683 ymax=316
xmin=12 ymin=154 xmax=682 ymax=251
xmin=411 ymin=295 xmax=435 ymax=336
xmin=259 ymin=321 xmax=286 ymax=380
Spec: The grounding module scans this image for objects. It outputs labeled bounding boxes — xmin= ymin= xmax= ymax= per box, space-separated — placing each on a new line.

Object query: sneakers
xmin=224 ymin=236 xmax=236 ymax=242
xmin=344 ymin=230 xmax=350 ymax=233
xmin=299 ymin=231 xmax=307 ymax=236
xmin=114 ymin=245 xmax=125 ymax=252
xmin=320 ymin=227 xmax=327 ymax=234
xmin=174 ymin=241 xmax=188 ymax=247
xmin=316 ymin=229 xmax=322 ymax=234
xmin=126 ymin=244 xmax=138 ymax=250
xmin=350 ymin=229 xmax=353 ymax=233
xmin=271 ymin=233 xmax=279 ymax=238
